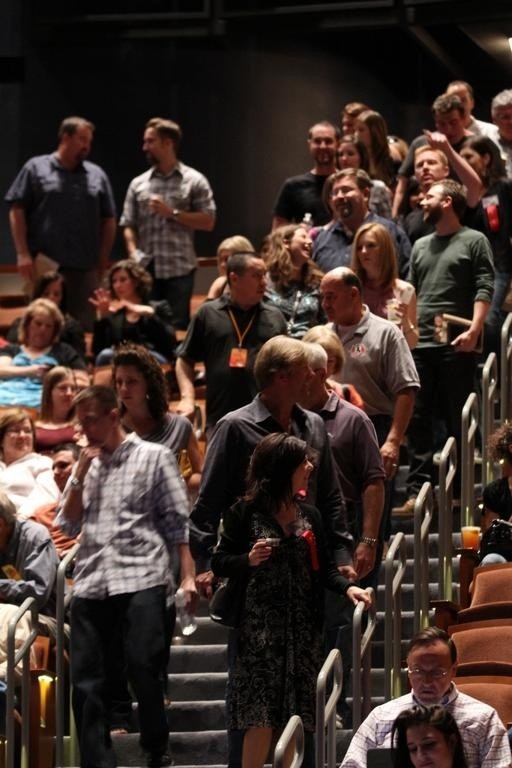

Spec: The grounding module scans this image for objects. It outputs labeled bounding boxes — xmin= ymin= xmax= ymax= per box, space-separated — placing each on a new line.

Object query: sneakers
xmin=389 ymin=493 xmax=474 ymax=518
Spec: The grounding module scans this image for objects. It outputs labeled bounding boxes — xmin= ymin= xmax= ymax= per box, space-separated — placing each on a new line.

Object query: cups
xmin=459 ymin=524 xmax=482 ymax=552
xmin=387 ymin=295 xmax=405 ymax=325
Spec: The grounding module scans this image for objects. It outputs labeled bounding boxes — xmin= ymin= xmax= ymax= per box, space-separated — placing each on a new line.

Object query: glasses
xmin=403 ymin=660 xmax=457 ymax=678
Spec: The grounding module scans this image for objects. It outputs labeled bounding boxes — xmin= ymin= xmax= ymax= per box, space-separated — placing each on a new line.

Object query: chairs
xmin=0 ymin=249 xmax=222 ymax=768
xmin=424 ymin=458 xmax=511 ymax=734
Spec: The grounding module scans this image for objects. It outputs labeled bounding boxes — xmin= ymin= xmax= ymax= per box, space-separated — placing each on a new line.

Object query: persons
xmin=2 ymin=81 xmax=510 ymax=766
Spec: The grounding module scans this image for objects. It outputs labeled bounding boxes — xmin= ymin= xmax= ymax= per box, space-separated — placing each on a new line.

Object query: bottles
xmin=172 ymin=588 xmax=198 ymax=636
